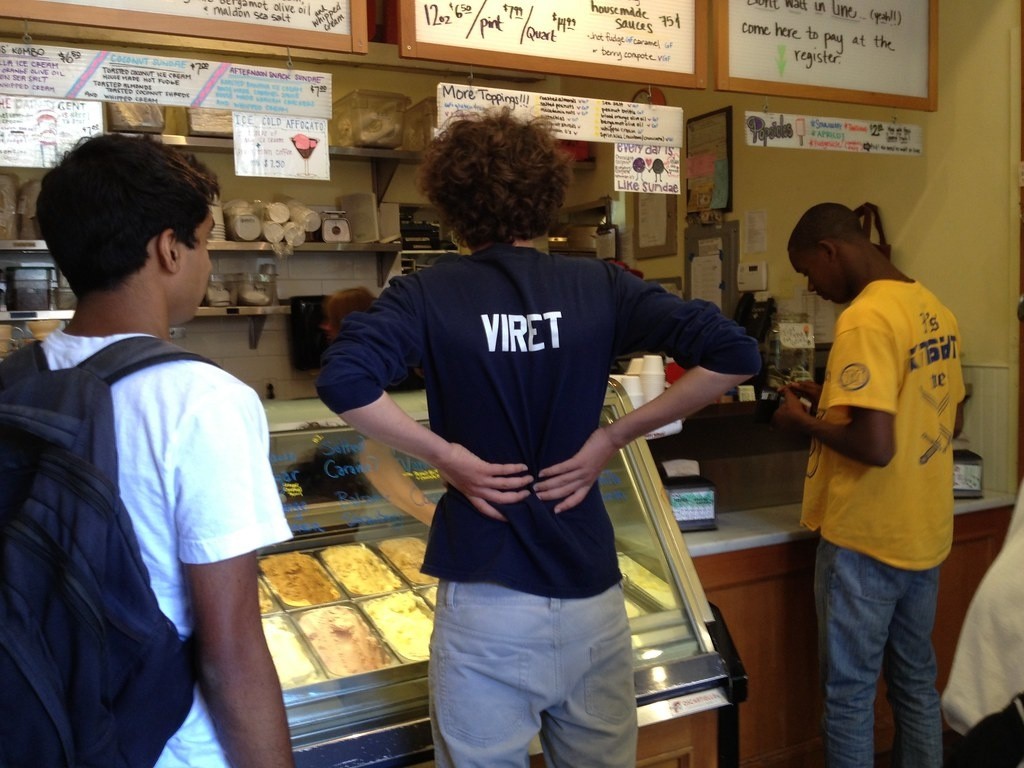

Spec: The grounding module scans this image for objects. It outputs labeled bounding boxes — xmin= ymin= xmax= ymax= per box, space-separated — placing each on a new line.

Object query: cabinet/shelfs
xmin=0 ymin=240 xmax=403 ymax=350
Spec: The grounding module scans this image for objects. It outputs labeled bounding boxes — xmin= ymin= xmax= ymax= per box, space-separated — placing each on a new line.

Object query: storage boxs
xmin=404 ymin=95 xmax=437 ymax=147
xmin=104 ymin=102 xmax=168 ymax=134
xmin=185 ymin=107 xmax=233 ymax=138
xmin=327 ymin=88 xmax=412 ymax=148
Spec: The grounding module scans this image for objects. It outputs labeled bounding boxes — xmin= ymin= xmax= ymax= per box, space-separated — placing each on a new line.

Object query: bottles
xmin=207 ymin=273 xmax=231 ymax=307
xmin=227 ymin=274 xmax=273 ymax=306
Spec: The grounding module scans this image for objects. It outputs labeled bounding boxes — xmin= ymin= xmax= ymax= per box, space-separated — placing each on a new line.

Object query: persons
xmin=323 ymin=286 xmax=439 ymax=527
xmin=767 ymin=203 xmax=965 ymax=768
xmin=940 ymin=472 xmax=1023 ymax=767
xmin=1 ymin=134 xmax=293 ymax=768
xmin=313 ymin=117 xmax=761 ymax=768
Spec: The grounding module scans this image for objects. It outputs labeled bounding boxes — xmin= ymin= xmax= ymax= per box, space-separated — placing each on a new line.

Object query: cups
xmin=263 ymin=203 xmax=289 ymax=224
xmin=223 ymin=199 xmax=262 ymax=241
xmin=261 ymin=218 xmax=284 ymax=242
xmin=282 ymin=222 xmax=307 ymax=247
xmin=625 ymin=358 xmax=643 ymax=375
xmin=206 ymin=194 xmax=226 ymax=242
xmin=283 ymin=197 xmax=321 ymax=232
xmin=621 ymin=377 xmax=644 ymax=410
xmin=641 ymin=355 xmax=666 ymax=403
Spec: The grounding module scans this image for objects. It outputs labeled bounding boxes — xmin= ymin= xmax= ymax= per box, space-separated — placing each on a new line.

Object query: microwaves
xmin=0 ymin=248 xmax=78 ymax=321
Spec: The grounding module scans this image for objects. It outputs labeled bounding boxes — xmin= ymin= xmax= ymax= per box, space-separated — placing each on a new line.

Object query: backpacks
xmin=0 ymin=335 xmax=223 ymax=768
